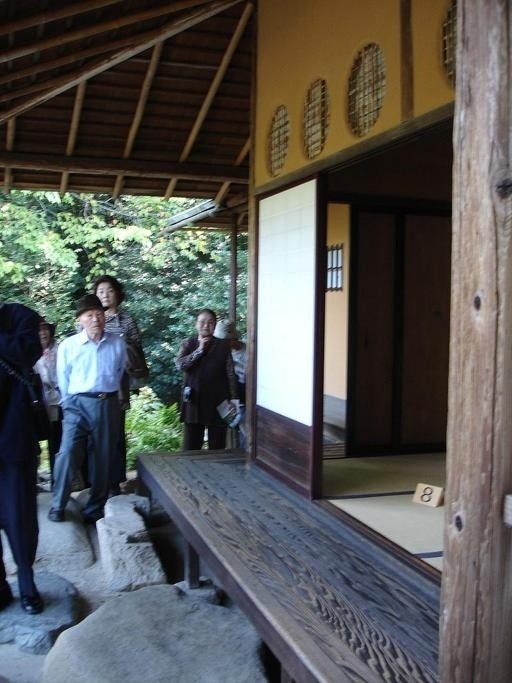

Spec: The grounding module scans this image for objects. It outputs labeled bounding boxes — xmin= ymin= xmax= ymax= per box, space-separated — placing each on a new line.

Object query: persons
xmin=32 ymin=312 xmax=66 ymax=493
xmin=211 ymin=317 xmax=247 ymax=450
xmin=1 ymin=301 xmax=54 ymax=614
xmin=76 ymin=274 xmax=149 ymax=497
xmin=48 ymin=294 xmax=140 ymax=523
xmin=175 ymin=308 xmax=241 ymax=451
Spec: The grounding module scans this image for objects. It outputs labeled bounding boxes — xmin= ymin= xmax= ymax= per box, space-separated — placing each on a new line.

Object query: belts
xmin=78 ymin=391 xmax=118 ymax=399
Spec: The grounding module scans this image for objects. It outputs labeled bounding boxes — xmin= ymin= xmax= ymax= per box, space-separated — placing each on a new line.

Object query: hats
xmin=214 ymin=319 xmax=241 ymax=338
xmin=39 ymin=316 xmax=55 ymax=337
xmin=76 ymin=294 xmax=109 ymax=317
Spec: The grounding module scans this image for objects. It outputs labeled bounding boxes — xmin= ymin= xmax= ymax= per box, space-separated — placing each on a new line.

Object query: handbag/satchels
xmin=217 ymin=399 xmax=236 ymax=424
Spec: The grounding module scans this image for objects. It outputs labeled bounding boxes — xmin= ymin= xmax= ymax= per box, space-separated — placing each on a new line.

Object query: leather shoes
xmin=48 ymin=507 xmax=64 ymax=521
xmin=0 ymin=582 xmax=43 ymax=614
